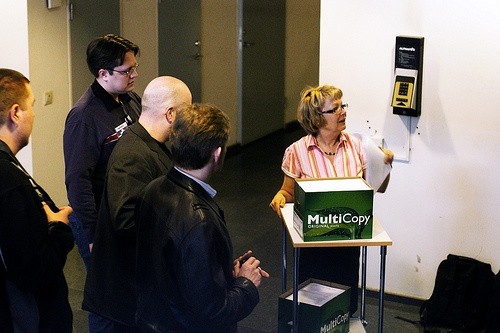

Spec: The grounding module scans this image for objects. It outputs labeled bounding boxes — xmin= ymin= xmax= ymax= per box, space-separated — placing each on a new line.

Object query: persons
xmin=134 ymin=104 xmax=270 ymax=333
xmin=0 ymin=68 xmax=73 ymax=333
xmin=269 ymin=85 xmax=394 ymax=319
xmin=80 ymin=73 xmax=192 ymax=333
xmin=62 ymin=33 xmax=143 ymax=273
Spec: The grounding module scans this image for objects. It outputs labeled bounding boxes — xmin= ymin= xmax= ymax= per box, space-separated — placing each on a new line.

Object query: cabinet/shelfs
xmin=280 ymin=202 xmax=392 ymax=333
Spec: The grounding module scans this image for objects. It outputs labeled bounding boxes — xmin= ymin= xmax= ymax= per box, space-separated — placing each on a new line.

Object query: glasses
xmin=109 ymin=64 xmax=139 ymax=76
xmin=320 ymin=104 xmax=348 ymax=114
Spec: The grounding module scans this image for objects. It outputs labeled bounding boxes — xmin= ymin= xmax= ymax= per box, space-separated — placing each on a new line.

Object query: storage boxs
xmin=293 ymin=176 xmax=374 ymax=242
xmin=277 ymin=278 xmax=352 ymax=333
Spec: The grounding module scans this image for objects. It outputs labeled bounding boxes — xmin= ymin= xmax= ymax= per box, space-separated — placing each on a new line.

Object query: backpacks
xmin=419 ymin=254 xmax=497 ymax=326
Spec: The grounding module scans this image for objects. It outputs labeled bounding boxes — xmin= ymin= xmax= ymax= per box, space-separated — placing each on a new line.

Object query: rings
xmin=257 ymin=267 xmax=261 ymax=270
